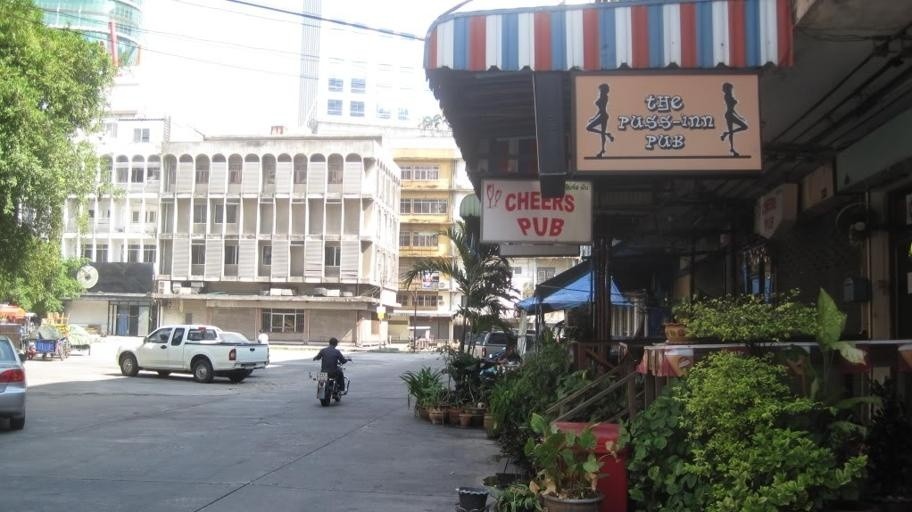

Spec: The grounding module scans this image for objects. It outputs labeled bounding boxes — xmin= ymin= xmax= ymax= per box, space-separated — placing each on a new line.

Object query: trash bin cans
xmin=546 ymin=422 xmax=628 ymax=512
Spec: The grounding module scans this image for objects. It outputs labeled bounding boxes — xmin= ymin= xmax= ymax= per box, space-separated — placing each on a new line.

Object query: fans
xmin=833 ymin=203 xmax=882 ymax=257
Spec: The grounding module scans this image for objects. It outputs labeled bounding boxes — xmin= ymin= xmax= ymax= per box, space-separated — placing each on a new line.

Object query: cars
xmin=0 ymin=335 xmax=26 ymax=430
xmin=188 ymin=331 xmax=249 ymax=343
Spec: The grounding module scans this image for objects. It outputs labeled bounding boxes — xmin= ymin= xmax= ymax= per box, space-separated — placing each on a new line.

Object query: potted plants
xmin=403 ymin=211 xmax=606 ymax=511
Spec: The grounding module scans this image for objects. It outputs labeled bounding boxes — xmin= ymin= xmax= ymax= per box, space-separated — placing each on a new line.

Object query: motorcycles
xmin=309 ymin=357 xmax=352 ymax=407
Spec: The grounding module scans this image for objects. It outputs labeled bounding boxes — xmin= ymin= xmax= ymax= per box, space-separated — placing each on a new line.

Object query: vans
xmin=481 ymin=330 xmax=536 ymax=367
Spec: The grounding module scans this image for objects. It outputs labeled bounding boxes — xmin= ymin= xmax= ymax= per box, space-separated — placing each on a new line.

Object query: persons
xmin=313 ymin=335 xmax=351 ymax=396
xmin=255 ymin=326 xmax=271 ymax=350
xmin=584 ymin=82 xmax=616 ymax=156
xmin=719 ymin=82 xmax=749 ymax=155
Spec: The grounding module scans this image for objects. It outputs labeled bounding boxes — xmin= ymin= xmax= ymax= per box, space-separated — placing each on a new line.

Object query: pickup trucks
xmin=116 ymin=324 xmax=270 ymax=383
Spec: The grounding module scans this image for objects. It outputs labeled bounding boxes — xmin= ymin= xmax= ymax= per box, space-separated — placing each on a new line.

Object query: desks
xmin=637 ymin=339 xmax=911 ymax=427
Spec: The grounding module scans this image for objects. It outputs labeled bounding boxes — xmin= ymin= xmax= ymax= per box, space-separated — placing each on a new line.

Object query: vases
xmin=662 ymin=321 xmax=695 ymax=345
